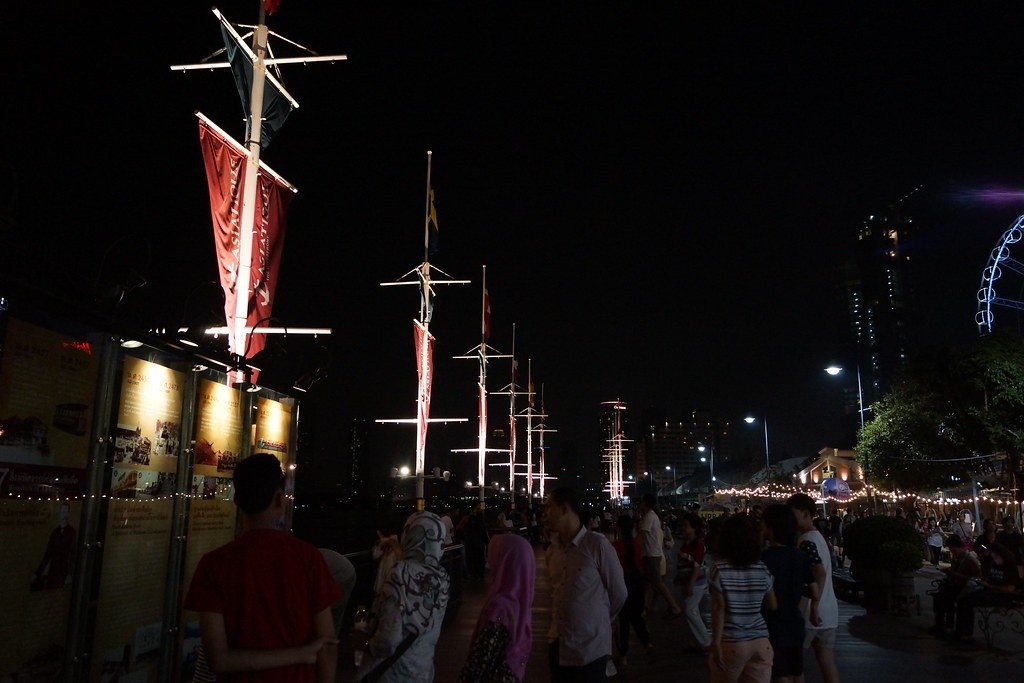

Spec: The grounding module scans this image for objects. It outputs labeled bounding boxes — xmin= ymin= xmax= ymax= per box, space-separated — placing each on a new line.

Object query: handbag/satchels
xmin=989 ymin=565 xmax=1008 ymax=586
xmin=337 ymin=605 xmax=418 ymax=683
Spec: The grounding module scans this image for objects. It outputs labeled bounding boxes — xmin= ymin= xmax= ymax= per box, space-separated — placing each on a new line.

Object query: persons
xmin=456 ymin=533 xmax=536 ymax=683
xmin=318 ymin=549 xmax=356 ymax=633
xmin=545 ymin=474 xmax=627 ymax=683
xmin=788 ymin=493 xmax=840 ymax=683
xmin=895 ymin=506 xmax=1024 ymax=635
xmin=612 ymin=514 xmax=656 ymax=666
xmin=675 ymin=514 xmax=711 ymax=652
xmin=184 ymin=454 xmax=339 ymax=683
xmin=352 ymin=511 xmax=453 ymax=683
xmin=610 ymin=503 xmax=675 ymax=548
xmin=708 ymin=516 xmax=777 ymax=683
xmin=721 ymin=505 xmax=762 ymax=522
xmin=755 ymin=505 xmax=819 ymax=683
xmin=494 ymin=507 xmax=613 ymax=541
xmin=638 ymin=494 xmax=682 ymax=624
xmin=690 ymin=503 xmax=702 ymax=514
xmin=814 ymin=508 xmax=854 ymax=546
xmin=32 ymin=502 xmax=77 ymax=589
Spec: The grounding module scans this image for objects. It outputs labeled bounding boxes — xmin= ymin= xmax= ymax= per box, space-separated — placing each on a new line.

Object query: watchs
xmin=359 ymin=639 xmax=368 ymax=649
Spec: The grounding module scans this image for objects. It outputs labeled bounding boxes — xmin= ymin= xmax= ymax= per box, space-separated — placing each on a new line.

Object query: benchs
xmin=925 ymin=569 xmax=1023 ymax=649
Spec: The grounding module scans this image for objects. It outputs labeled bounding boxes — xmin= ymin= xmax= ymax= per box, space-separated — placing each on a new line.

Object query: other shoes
xmin=651 ymin=646 xmax=664 ymax=661
xmin=617 ymin=658 xmax=633 ymax=664
xmin=930 ymin=626 xmax=966 ymax=636
